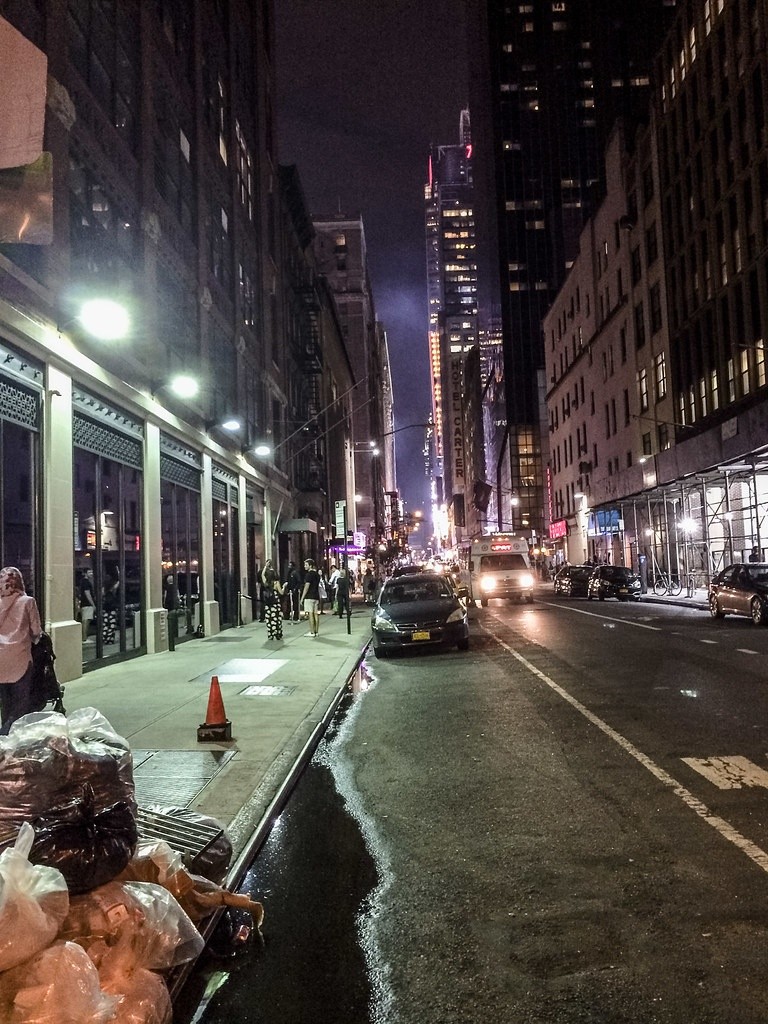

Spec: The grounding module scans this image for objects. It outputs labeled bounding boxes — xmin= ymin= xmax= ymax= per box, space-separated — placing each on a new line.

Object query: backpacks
xmin=263 ymin=587 xmax=276 ymax=606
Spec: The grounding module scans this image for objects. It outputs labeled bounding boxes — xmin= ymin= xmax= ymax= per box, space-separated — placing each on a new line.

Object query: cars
xmin=706 ymin=562 xmax=768 ymax=626
xmin=370 ymin=570 xmax=471 ymax=660
xmin=586 ymin=564 xmax=643 ymax=603
xmin=553 ymin=564 xmax=595 ymax=597
xmin=383 ymin=560 xmax=469 ymax=599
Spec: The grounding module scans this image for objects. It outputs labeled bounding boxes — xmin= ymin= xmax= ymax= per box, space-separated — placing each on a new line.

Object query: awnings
xmin=279 ymin=518 xmax=317 ymax=534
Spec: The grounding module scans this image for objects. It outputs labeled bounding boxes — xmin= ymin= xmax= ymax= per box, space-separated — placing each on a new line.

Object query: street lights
xmin=427 ymin=547 xmax=433 ymax=558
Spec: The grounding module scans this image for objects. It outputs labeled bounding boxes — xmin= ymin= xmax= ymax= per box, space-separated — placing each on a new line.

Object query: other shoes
xmin=304 ymin=631 xmax=319 ymax=637
xmin=320 ymin=612 xmax=326 ymax=614
xmin=332 ymin=611 xmax=339 ymax=615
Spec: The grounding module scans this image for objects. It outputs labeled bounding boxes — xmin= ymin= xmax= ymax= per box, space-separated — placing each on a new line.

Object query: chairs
xmin=427 ymin=584 xmax=438 ymax=596
xmin=393 ymin=588 xmax=405 ymax=597
xmin=739 ymin=572 xmax=750 ymax=584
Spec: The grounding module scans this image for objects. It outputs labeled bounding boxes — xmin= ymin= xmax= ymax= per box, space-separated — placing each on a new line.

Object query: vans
xmin=468 ymin=532 xmax=539 ymax=607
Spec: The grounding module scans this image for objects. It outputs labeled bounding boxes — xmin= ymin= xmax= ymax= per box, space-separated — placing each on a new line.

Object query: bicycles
xmin=653 ymin=572 xmax=682 ymax=596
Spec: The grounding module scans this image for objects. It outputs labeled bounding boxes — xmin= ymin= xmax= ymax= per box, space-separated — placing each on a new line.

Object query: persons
xmin=638 ymin=554 xmax=646 ymax=570
xmin=559 ymin=560 xmax=571 ymax=570
xmin=0 ymin=567 xmax=42 ymax=737
xmin=163 ymin=575 xmax=181 ymax=610
xmin=584 ymin=555 xmax=610 ymax=567
xmin=537 ymin=562 xmax=553 ymax=582
xmin=80 ymin=567 xmax=96 ymax=643
xmin=102 ymin=566 xmax=120 ymax=644
xmin=749 ymin=546 xmax=765 ymax=562
xmin=256 ymin=559 xmax=372 ymax=639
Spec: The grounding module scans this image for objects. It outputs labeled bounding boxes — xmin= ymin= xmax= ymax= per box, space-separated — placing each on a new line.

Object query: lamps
xmin=58 ymin=282 xmax=139 ymax=353
xmin=153 ymin=368 xmax=201 ymax=401
xmin=206 ymin=414 xmax=241 ymax=431
xmin=243 ymin=443 xmax=271 ymax=458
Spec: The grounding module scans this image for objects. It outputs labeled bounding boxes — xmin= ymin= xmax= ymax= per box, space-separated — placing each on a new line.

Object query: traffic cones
xmin=196 ymin=676 xmax=232 ymax=743
